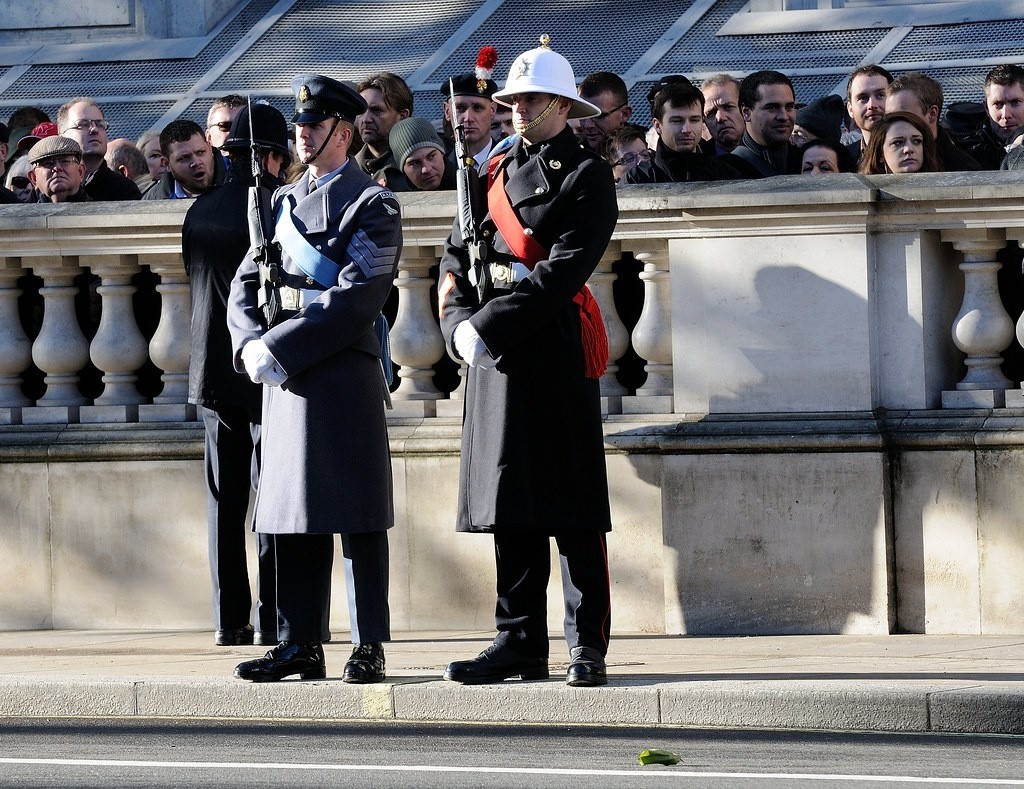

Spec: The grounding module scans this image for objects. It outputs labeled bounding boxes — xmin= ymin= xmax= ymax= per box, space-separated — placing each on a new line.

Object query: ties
xmin=466 ymin=157 xmax=475 ymax=166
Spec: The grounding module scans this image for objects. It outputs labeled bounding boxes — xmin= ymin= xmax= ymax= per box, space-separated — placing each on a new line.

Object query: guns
xmin=246 ymin=95 xmax=284 ymax=330
xmin=449 ymin=75 xmax=493 ymax=305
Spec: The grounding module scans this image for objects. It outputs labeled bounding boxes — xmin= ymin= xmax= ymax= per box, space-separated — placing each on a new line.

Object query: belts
xmin=486 ymin=261 xmax=534 ymax=285
xmin=278 ymin=286 xmax=324 ymax=311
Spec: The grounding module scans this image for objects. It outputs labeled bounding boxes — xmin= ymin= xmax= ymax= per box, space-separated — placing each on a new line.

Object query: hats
xmin=26 ymin=135 xmax=82 ymax=165
xmin=218 ymin=104 xmax=292 ymax=170
xmin=793 ymin=94 xmax=846 ymax=143
xmin=493 ymin=36 xmax=600 ymax=121
xmin=16 ymin=121 xmax=58 ymax=151
xmin=290 ymin=73 xmax=367 ymax=126
xmin=439 ymin=45 xmax=498 ymax=101
xmin=389 ymin=117 xmax=445 ymax=172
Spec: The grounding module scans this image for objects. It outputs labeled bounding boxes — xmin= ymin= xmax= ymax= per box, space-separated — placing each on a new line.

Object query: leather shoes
xmin=232 ymin=641 xmax=327 ymax=680
xmin=214 ymin=624 xmax=255 ymax=645
xmin=443 ymin=641 xmax=550 ymax=684
xmin=566 ymin=646 xmax=607 ymax=686
xmin=343 ymin=642 xmax=386 ymax=683
xmin=256 ymin=632 xmax=280 ymax=645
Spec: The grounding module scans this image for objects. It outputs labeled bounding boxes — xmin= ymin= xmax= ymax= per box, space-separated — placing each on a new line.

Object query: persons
xmin=181 ymin=106 xmax=291 ymax=646
xmin=226 ymin=74 xmax=403 ymax=683
xmin=0 ymin=65 xmax=1024 ymax=403
xmin=437 ymin=35 xmax=619 ymax=686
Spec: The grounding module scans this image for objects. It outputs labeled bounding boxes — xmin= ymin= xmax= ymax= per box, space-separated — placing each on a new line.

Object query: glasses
xmin=33 ymin=154 xmax=79 ymax=170
xmin=611 ymin=148 xmax=654 ymax=169
xmin=208 ymin=121 xmax=234 ymax=132
xmin=596 ymin=101 xmax=630 ymax=120
xmin=61 ymin=118 xmax=110 ymax=136
xmin=11 ymin=176 xmax=30 ymax=188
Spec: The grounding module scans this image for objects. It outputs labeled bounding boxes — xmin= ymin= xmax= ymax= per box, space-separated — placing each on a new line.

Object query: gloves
xmin=452 ymin=321 xmax=502 ymax=371
xmin=241 ymin=339 xmax=274 ymax=383
xmin=257 ymin=362 xmax=287 ymax=388
